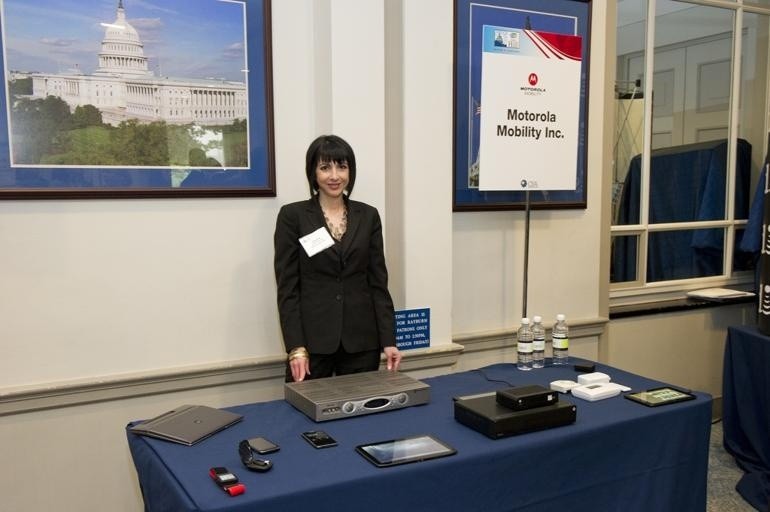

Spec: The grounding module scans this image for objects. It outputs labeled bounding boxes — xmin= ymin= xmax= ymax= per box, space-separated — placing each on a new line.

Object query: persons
xmin=272 ymin=133 xmax=404 ymax=385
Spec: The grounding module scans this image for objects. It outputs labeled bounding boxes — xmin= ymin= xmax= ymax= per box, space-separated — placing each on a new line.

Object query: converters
xmin=575 ymin=363 xmax=595 ymax=373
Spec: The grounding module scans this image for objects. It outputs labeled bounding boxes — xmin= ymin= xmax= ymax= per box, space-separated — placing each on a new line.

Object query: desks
xmin=722 ymin=324 xmax=769 ymax=511
xmin=125 ymin=356 xmax=714 ymax=511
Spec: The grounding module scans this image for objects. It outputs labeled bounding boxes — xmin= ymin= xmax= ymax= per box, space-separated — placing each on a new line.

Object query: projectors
xmin=283 ymin=367 xmax=432 ymax=422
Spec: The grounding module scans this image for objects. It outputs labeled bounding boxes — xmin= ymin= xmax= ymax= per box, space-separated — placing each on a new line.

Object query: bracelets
xmin=286 ymin=351 xmax=310 ymax=360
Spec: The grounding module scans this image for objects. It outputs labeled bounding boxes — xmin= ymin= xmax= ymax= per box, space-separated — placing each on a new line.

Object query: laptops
xmin=129 ymin=402 xmax=244 ymax=447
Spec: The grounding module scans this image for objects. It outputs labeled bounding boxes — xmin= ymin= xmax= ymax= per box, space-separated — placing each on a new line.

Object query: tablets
xmin=623 ymin=386 xmax=698 ymax=408
xmin=355 ymin=431 xmax=458 ymax=468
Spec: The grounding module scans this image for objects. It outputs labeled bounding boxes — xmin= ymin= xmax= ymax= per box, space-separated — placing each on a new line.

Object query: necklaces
xmin=319 ymin=203 xmax=349 ymax=241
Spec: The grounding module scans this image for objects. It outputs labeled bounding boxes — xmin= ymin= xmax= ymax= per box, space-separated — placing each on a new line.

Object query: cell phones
xmin=301 ymin=429 xmax=340 ymax=449
xmin=248 ymin=436 xmax=281 ymax=454
xmin=209 ymin=466 xmax=240 ymax=492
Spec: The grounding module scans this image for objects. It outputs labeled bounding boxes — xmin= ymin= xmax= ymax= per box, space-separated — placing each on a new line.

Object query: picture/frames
xmin=451 ymin=0 xmax=593 ymax=212
xmin=1 ymin=0 xmax=278 ymax=199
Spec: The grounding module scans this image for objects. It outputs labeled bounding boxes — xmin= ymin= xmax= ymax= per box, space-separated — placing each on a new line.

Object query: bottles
xmin=531 ymin=314 xmax=546 ymax=369
xmin=551 ymin=314 xmax=570 ymax=366
xmin=516 ymin=317 xmax=533 ymax=372
